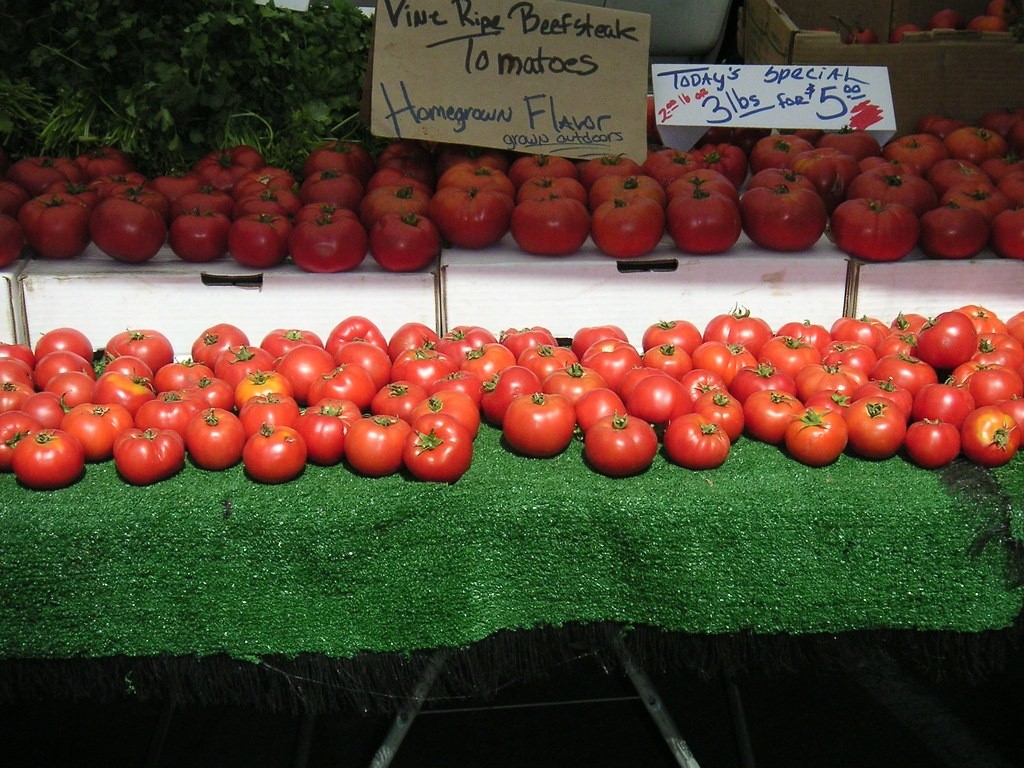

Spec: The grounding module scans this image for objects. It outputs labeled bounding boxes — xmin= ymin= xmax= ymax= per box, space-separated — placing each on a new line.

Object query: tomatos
xmin=0 ymin=96 xmax=1024 ymax=263
xmin=812 ymin=0 xmax=1024 ymax=44
xmin=0 ymin=302 xmax=1024 ymax=490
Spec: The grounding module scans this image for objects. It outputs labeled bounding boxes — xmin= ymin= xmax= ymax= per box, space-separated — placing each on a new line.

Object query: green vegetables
xmin=0 ymin=0 xmax=487 ymax=181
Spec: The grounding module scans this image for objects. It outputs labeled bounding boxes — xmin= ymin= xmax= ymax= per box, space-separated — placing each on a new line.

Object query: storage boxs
xmin=18 ymin=229 xmax=441 ymax=367
xmin=438 ymin=208 xmax=853 ymax=358
xmin=746 ymin=0 xmax=1024 ymax=130
xmin=1 ymin=248 xmax=33 ymax=349
xmin=581 ymin=0 xmax=734 ymax=58
xmin=852 ymin=210 xmax=1024 ymax=328
